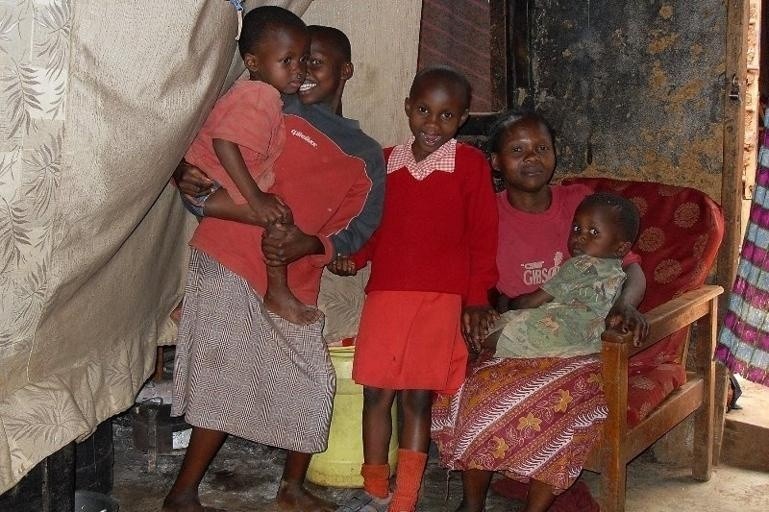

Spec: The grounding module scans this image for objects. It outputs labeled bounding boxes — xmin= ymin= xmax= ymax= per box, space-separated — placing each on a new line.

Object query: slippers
xmin=334 ymin=490 xmax=391 ymax=511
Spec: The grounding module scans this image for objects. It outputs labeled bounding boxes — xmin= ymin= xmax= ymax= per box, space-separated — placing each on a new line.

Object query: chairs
xmin=557 ymin=175 xmax=726 ymax=512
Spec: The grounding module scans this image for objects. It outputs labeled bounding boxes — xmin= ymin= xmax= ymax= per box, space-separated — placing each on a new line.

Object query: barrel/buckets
xmin=306 ymin=345 xmax=399 ymax=488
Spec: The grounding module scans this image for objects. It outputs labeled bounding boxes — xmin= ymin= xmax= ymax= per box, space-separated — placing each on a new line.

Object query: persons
xmin=161 ymin=23 xmax=387 ymax=511
xmin=426 ymin=110 xmax=653 ymax=512
xmin=323 ymin=63 xmax=500 ymax=512
xmin=167 ymin=4 xmax=323 ymax=328
xmin=461 ymin=190 xmax=640 ymax=361
